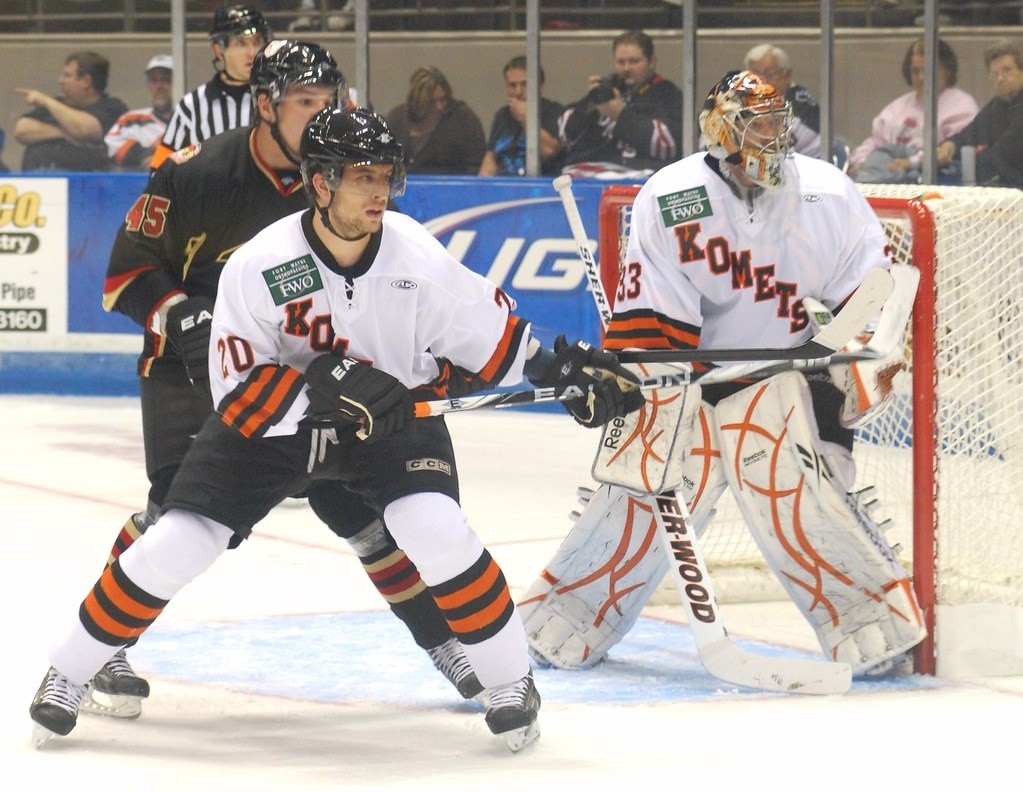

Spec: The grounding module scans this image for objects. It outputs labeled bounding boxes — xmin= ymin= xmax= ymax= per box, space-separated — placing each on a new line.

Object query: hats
xmin=144 ymin=55 xmax=173 ymax=72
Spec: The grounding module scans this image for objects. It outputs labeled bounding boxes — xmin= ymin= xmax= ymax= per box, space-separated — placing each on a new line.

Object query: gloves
xmin=167 ymin=300 xmax=216 ymax=397
xmin=544 ymin=334 xmax=646 ymax=428
xmin=303 ymin=353 xmax=416 ymax=444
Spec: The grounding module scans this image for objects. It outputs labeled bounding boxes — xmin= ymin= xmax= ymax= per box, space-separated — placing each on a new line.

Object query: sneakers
xmin=485 ymin=670 xmax=542 ymax=752
xmin=32 ymin=666 xmax=89 ymax=751
xmin=429 ymin=637 xmax=490 ymax=710
xmin=81 ymin=647 xmax=149 ymax=719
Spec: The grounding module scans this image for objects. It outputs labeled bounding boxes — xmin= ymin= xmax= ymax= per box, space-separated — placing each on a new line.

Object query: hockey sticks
xmin=551 ymin=169 xmax=855 ymax=697
xmin=614 ymin=266 xmax=895 ymax=365
xmin=413 ymin=260 xmax=923 ymax=419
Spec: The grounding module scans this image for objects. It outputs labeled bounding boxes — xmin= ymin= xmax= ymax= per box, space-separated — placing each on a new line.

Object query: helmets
xmin=699 ymin=75 xmax=792 ymax=194
xmin=209 ymin=4 xmax=266 ymax=43
xmin=247 ymin=36 xmax=344 ymax=105
xmin=298 ymin=106 xmax=404 ymax=169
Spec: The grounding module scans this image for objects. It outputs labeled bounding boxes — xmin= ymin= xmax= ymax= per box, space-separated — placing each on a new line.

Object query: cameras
xmin=587 ymin=74 xmax=623 ymax=102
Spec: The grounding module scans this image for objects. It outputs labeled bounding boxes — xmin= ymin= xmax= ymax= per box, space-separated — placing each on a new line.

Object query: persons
xmin=555 ymin=27 xmax=684 ymax=178
xmin=83 ymin=42 xmax=496 ymax=699
xmin=12 ymin=51 xmax=126 ymax=169
xmin=285 ymin=0 xmax=360 ymax=32
xmin=742 ymin=44 xmax=821 ymax=159
xmin=848 ymin=37 xmax=978 ymax=185
xmin=147 ymin=6 xmax=271 ymax=178
xmin=106 ymin=54 xmax=178 ymax=166
xmin=28 ymin=106 xmax=645 ymax=736
xmin=949 ymin=39 xmax=1023 ymax=188
xmin=478 ymin=61 xmax=565 ymax=176
xmin=512 ymin=71 xmax=927 ymax=679
xmin=385 ymin=66 xmax=485 ymax=176
